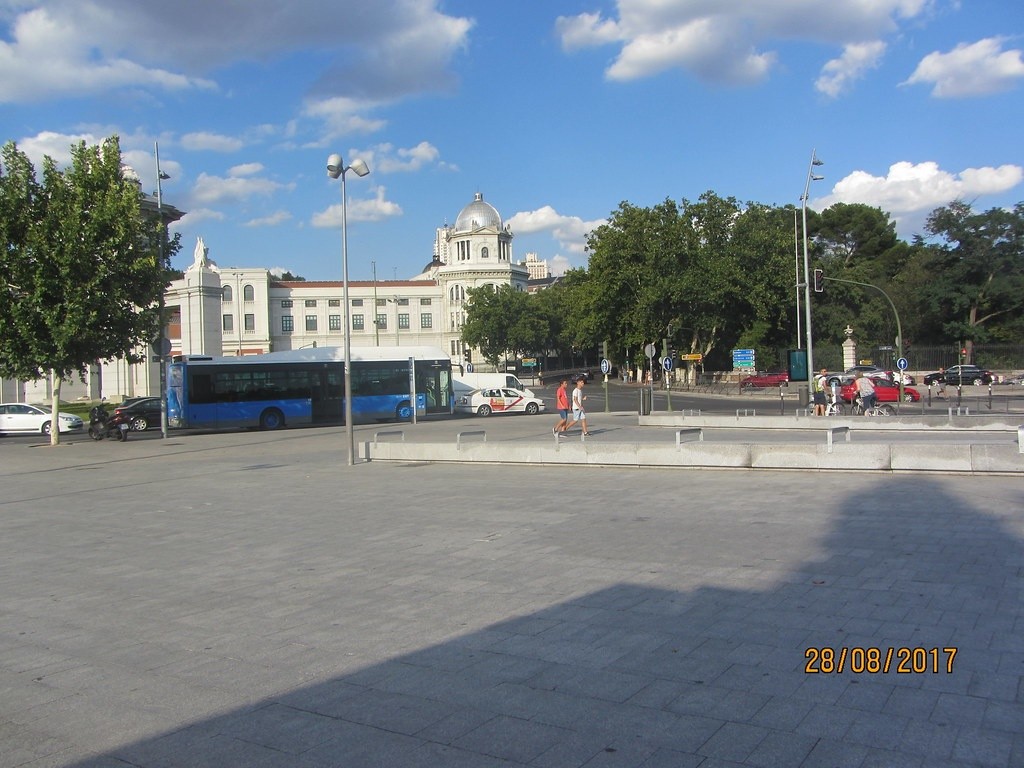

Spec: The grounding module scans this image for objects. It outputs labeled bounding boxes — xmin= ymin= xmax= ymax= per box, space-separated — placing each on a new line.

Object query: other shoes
xmin=553 ymin=427 xmax=558 ymax=436
xmin=584 ymin=432 xmax=592 ymax=437
xmin=559 ymin=430 xmax=567 ymax=437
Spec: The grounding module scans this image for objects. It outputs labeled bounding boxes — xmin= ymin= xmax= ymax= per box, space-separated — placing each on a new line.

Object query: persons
xmin=644 ymin=370 xmax=651 ymax=385
xmin=853 ymin=370 xmax=876 ymax=415
xmin=813 ymin=368 xmax=835 ymax=416
xmin=623 ymin=369 xmax=633 ymax=382
xmin=937 ymin=368 xmax=948 ymax=397
xmin=553 ymin=378 xmax=591 ymax=437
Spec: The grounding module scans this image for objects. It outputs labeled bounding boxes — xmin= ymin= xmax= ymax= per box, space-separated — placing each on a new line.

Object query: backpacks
xmin=812 ymin=376 xmax=824 ymax=393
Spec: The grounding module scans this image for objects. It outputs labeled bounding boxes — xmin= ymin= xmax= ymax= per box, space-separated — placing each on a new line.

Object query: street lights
xmin=371 ymin=261 xmax=379 ymax=346
xmin=154 ymin=140 xmax=171 ymax=440
xmin=782 ymin=207 xmax=802 ymax=349
xmin=325 ymin=153 xmax=370 ymax=466
xmin=232 ymin=272 xmax=244 ymax=357
xmin=801 ymin=147 xmax=824 ymax=396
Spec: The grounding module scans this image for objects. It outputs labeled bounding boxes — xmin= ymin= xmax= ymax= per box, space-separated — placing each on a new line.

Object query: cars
xmin=113 ymin=397 xmax=162 ymax=433
xmin=740 ymin=370 xmax=790 ymax=388
xmin=826 ymin=364 xmax=915 ymax=387
xmin=0 ymin=402 xmax=83 ymax=436
xmin=570 ymin=368 xmax=592 ymax=384
xmin=841 ymin=376 xmax=920 ymax=403
xmin=1001 ymin=373 xmax=1024 ymax=385
xmin=924 ymin=363 xmax=992 ymax=386
xmin=454 ymin=388 xmax=546 ymax=417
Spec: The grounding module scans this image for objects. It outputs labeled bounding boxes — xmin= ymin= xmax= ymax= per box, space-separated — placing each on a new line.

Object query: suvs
xmin=120 ymin=396 xmax=150 ymax=407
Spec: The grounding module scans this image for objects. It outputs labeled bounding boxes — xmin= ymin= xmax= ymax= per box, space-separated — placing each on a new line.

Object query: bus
xmin=164 ymin=346 xmax=465 ymax=431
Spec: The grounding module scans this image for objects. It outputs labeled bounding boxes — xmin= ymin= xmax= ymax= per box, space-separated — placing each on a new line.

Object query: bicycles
xmin=850 ymin=390 xmax=897 ymax=416
xmin=806 ymin=393 xmax=846 ymax=417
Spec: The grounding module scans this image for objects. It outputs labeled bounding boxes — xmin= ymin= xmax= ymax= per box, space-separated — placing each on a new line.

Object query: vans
xmin=440 ymin=372 xmax=536 ymax=408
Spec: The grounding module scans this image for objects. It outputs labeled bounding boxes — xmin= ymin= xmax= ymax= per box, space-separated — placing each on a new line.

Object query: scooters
xmin=85 ymin=397 xmax=130 ymax=442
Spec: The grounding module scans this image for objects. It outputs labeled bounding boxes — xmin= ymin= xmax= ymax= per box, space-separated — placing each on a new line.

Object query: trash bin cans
xmin=638 ymin=387 xmax=651 ymax=415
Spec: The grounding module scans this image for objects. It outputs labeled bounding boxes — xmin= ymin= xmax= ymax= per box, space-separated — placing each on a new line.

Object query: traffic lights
xmin=662 ymin=338 xmax=673 ymax=356
xmin=667 ymin=323 xmax=674 ymax=336
xmin=598 ymin=340 xmax=607 ymax=359
xmin=813 ymin=268 xmax=823 ymax=292
xmin=464 ymin=350 xmax=471 ymax=364
xmin=904 ymin=339 xmax=912 ymax=355
xmin=955 ymin=340 xmax=961 ymax=353
xmin=671 ymin=349 xmax=677 ymax=360
xmin=960 ymin=346 xmax=967 ymax=359
